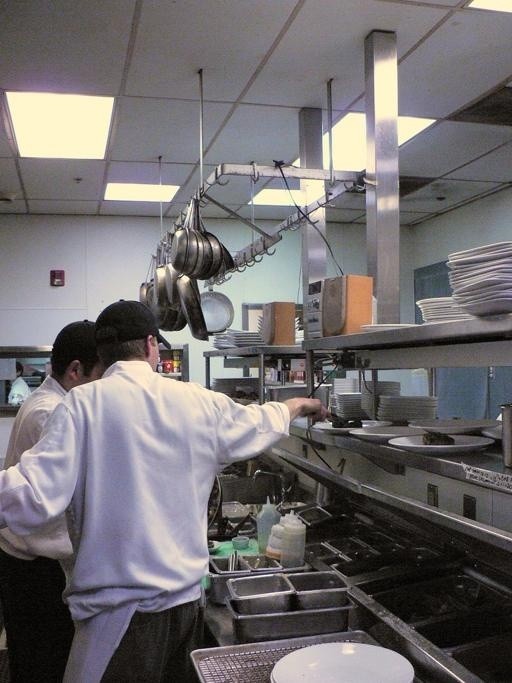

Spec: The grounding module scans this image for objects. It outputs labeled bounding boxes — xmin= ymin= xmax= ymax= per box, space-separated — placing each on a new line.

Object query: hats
xmin=52 ymin=319 xmax=97 ymax=358
xmin=93 ymin=299 xmax=171 ymax=350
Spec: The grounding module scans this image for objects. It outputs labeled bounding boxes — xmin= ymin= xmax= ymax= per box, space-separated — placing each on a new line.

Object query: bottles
xmin=256 ymin=495 xmax=306 ymax=569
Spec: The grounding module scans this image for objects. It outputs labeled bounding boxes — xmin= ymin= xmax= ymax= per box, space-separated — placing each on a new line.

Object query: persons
xmin=6 ymin=359 xmax=32 ymax=405
xmin=0 ymin=297 xmax=330 ymax=682
xmin=0 ymin=317 xmax=106 ymax=682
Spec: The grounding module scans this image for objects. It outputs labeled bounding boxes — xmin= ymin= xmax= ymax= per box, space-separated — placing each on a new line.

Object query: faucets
xmin=250 ymin=470 xmax=296 ymax=506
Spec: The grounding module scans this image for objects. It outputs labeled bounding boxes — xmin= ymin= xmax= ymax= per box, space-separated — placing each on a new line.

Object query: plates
xmin=212 ymin=327 xmax=267 ymax=350
xmin=270 ymin=642 xmax=415 ymax=683
xmin=360 ymin=241 xmax=512 ymax=333
xmin=312 ymin=378 xmax=504 ymax=455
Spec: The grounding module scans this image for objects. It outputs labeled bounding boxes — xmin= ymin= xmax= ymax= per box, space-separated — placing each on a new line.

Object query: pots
xmin=139 ymin=198 xmax=235 ymax=342
